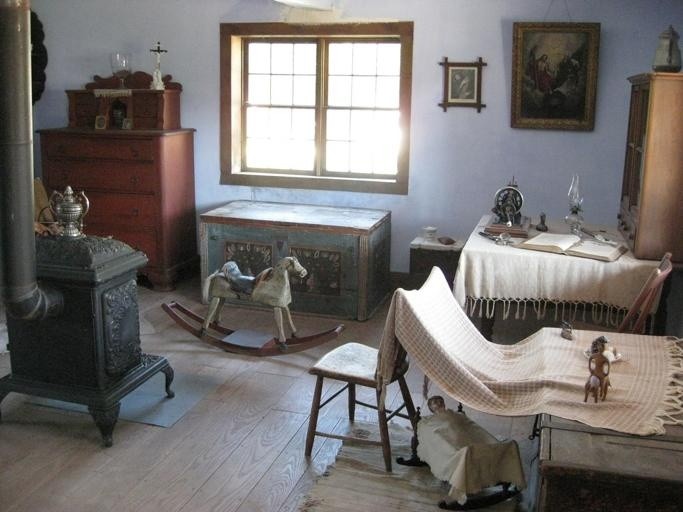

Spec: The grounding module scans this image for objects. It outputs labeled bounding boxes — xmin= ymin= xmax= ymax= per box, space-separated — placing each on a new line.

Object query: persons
xmin=452 ymin=72 xmax=473 ymax=99
xmin=589 ymin=336 xmax=610 ymax=396
xmin=428 ymin=395 xmax=445 ymax=413
xmin=537 ymin=55 xmax=553 ymax=95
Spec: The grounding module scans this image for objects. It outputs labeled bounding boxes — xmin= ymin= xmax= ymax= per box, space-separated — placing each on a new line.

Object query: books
xmin=517 ymin=232 xmax=628 ymax=262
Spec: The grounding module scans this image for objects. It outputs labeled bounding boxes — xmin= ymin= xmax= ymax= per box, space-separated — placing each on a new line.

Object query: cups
xmin=423 ymin=226 xmax=437 ymax=242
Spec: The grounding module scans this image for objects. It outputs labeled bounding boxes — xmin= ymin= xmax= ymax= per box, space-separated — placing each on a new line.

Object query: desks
xmin=528 ymin=327 xmax=683 ymax=510
xmin=455 ymin=217 xmax=683 ymax=343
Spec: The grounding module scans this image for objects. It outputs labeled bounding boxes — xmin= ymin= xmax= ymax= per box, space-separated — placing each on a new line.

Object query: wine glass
xmin=108 ymin=50 xmax=133 ymax=89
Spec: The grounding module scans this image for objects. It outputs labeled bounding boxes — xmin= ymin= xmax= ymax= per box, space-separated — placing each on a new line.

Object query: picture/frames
xmin=511 ymin=22 xmax=601 ymax=133
xmin=437 ymin=57 xmax=487 ymax=114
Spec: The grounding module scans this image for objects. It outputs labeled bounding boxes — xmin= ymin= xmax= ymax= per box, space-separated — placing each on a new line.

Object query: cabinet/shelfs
xmin=64 ymin=71 xmax=183 ymax=130
xmin=407 ymin=236 xmax=465 ymax=294
xmin=36 ymin=128 xmax=194 ymax=292
xmin=198 ymin=200 xmax=391 ymax=321
xmin=616 ymin=72 xmax=683 ymax=263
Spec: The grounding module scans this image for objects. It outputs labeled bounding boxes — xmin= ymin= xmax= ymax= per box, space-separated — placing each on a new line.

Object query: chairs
xmin=615 ymin=251 xmax=673 ymax=334
xmin=304 ymin=265 xmax=442 ymax=475
